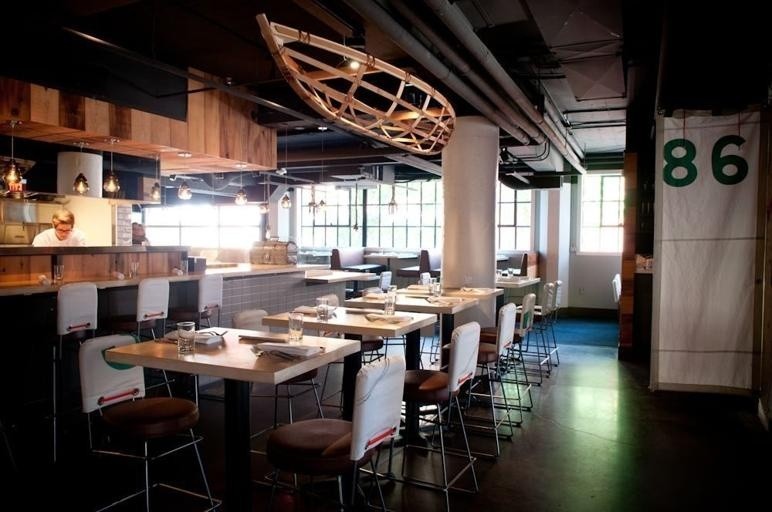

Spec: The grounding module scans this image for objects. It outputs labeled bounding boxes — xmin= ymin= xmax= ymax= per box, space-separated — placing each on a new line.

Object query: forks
xmin=196 ymin=330 xmax=228 ymax=336
xmin=250 ymin=345 xmax=300 ymax=361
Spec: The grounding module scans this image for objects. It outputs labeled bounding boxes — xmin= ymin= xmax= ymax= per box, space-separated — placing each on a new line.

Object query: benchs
xmin=397 ymin=248 xmax=424 ymax=277
xmin=422 ymin=247 xmax=441 ymax=277
xmin=331 ymin=246 xmax=383 ymax=300
xmin=504 ymin=253 xmax=538 ymax=277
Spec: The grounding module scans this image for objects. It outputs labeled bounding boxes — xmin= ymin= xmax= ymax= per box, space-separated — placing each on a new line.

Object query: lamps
xmin=3 ymin=122 xmax=293 ymax=210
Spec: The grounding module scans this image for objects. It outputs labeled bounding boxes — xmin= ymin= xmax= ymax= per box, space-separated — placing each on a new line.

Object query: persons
xmin=32 ymin=208 xmax=87 ymax=247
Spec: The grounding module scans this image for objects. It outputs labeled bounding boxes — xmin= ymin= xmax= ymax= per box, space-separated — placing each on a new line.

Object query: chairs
xmin=611 ymin=272 xmax=621 ymax=316
xmin=260 ymin=355 xmax=406 ymax=511
xmin=103 ymin=276 xmax=176 ymax=401
xmin=169 ymin=273 xmax=223 ymax=408
xmin=399 ymin=319 xmax=480 ymax=511
xmin=78 ymin=333 xmax=222 ymax=512
xmin=36 ymin=282 xmax=99 ymax=465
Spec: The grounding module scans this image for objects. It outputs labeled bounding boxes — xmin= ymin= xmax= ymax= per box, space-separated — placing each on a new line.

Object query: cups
xmin=433 ymin=283 xmax=441 ymax=297
xmin=180 ymin=258 xmax=206 ymax=275
xmin=177 ymin=321 xmax=195 ymax=357
xmin=384 ymin=295 xmax=395 ymax=316
xmin=430 ymin=278 xmax=437 ymax=284
xmin=316 ymin=297 xmax=328 ymax=322
xmin=508 ymin=268 xmax=513 ymax=279
xmin=129 ymin=263 xmax=137 ymax=280
xmin=387 ymin=285 xmax=397 ymax=297
xmin=289 ymin=313 xmax=304 ymax=341
xmin=54 ymin=264 xmax=64 ymax=288
xmin=497 ymin=270 xmax=502 ymax=280
